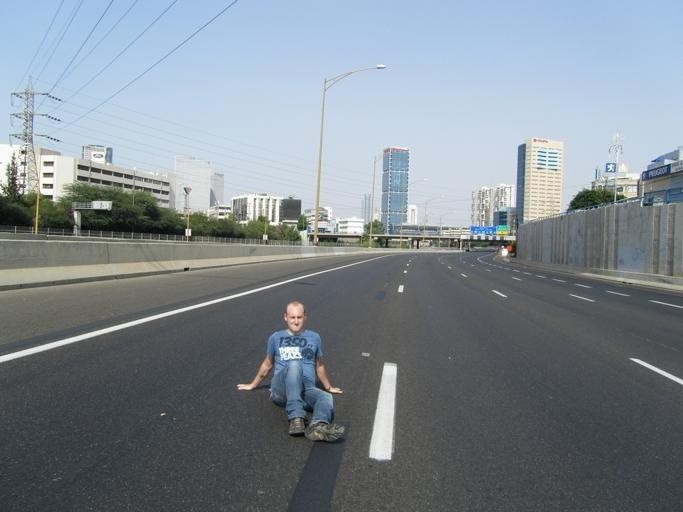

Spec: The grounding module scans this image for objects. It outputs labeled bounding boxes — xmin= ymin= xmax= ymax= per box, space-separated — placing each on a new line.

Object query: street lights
xmin=399 ymin=177 xmax=428 ymax=248
xmin=312 ymin=64 xmax=386 ymax=245
xmin=367 ymin=152 xmax=393 ymax=247
xmin=422 ymin=195 xmax=445 ymax=249
xmin=608 ymin=128 xmax=624 ymax=206
xmin=438 ymin=210 xmax=455 ymax=249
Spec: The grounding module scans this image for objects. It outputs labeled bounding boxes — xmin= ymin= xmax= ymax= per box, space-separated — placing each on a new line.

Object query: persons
xmin=236 ymin=300 xmax=345 ymax=442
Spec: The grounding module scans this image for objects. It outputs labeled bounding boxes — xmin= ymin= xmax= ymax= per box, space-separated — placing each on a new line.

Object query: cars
xmin=418 ymin=239 xmax=432 ymax=248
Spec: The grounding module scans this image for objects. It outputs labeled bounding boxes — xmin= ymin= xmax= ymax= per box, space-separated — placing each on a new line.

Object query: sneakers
xmin=288 ymin=417 xmax=305 ymax=435
xmin=304 ymin=422 xmax=344 ymax=442
